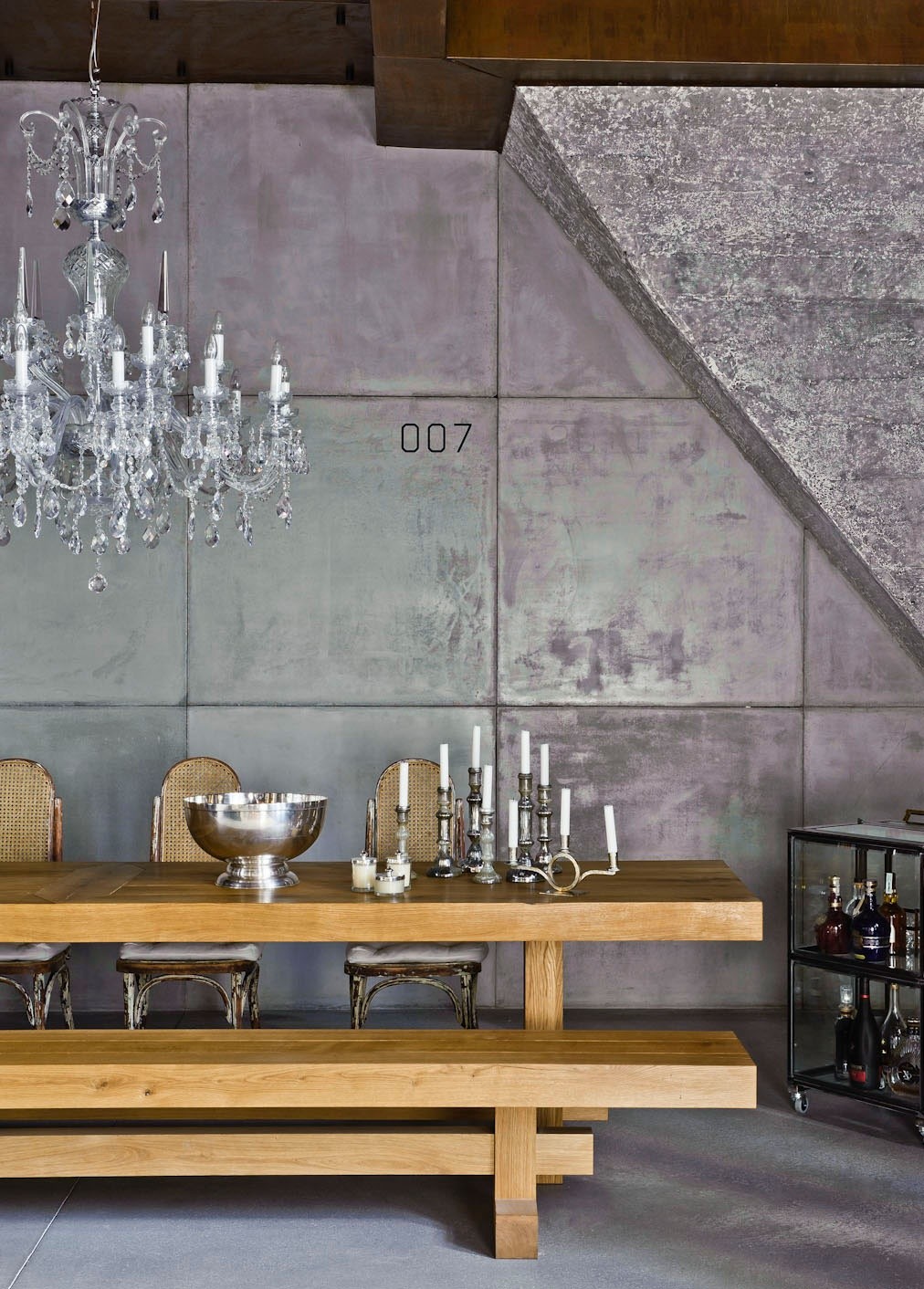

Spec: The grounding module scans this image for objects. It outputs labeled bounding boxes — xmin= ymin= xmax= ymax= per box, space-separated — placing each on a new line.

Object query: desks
xmin=0 ymin=854 xmax=764 ymax=1031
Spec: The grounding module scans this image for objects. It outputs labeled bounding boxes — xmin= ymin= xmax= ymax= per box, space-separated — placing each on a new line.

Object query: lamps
xmin=0 ymin=0 xmax=312 ymax=596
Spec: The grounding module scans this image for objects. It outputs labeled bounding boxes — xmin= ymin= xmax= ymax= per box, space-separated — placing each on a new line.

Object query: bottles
xmin=350 ymin=850 xmax=377 ymax=893
xmin=814 ymin=875 xmax=852 ymax=954
xmin=879 ymin=983 xmax=908 ymax=1086
xmin=374 ymin=869 xmax=405 ymax=897
xmin=847 ymin=977 xmax=880 ymax=1090
xmin=844 ymin=877 xmax=891 ymax=963
xmin=887 ymin=1016 xmax=921 ymax=1098
xmin=834 ymin=985 xmax=856 ymax=1080
xmin=877 ymin=872 xmax=907 ymax=956
xmin=385 ymin=849 xmax=411 ymax=887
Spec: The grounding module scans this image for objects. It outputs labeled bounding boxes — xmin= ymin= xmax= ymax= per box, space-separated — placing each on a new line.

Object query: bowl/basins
xmin=182 ymin=791 xmax=328 ymax=889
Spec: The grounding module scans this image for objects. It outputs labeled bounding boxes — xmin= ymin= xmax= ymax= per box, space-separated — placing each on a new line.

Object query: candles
xmin=396 ymin=760 xmax=411 ymax=809
xmin=482 ymin=764 xmax=492 ymax=810
xmin=519 ymin=729 xmax=533 ymax=776
xmin=471 ymin=722 xmax=481 ymax=768
xmin=508 ymin=797 xmax=518 ymax=848
xmin=386 ymin=850 xmax=411 ymax=889
xmin=539 ymin=741 xmax=551 ymax=788
xmin=351 ymin=852 xmax=378 ymax=893
xmin=560 ymin=783 xmax=571 ymax=837
xmin=373 ymin=867 xmax=405 ymax=895
xmin=603 ymin=803 xmax=619 ymax=854
xmin=439 ymin=741 xmax=449 ymax=789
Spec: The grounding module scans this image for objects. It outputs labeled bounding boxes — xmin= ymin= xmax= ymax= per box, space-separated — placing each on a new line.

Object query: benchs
xmin=0 ymin=1025 xmax=760 ymax=1261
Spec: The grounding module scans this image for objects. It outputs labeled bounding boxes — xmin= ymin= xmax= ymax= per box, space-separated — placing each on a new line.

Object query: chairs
xmin=115 ymin=755 xmax=263 ymax=1030
xmin=0 ymin=753 xmax=76 ymax=1030
xmin=345 ymin=756 xmax=489 ymax=1030
